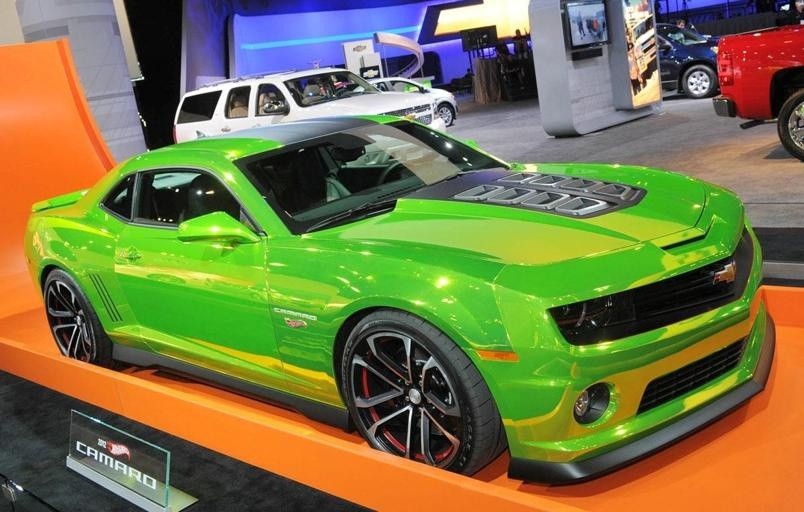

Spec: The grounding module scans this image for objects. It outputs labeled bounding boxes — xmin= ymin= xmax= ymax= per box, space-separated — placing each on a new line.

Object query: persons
xmin=576 ymin=12 xmax=605 ymax=40
xmin=513 ymin=28 xmax=528 ymax=60
xmin=675 ymin=19 xmax=685 ymax=29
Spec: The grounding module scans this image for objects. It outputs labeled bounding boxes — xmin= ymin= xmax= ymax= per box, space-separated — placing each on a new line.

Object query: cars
xmin=335 ymin=74 xmax=459 ymax=129
xmin=653 ymin=18 xmax=722 ymax=101
xmin=623 ymin=16 xmax=646 ymax=96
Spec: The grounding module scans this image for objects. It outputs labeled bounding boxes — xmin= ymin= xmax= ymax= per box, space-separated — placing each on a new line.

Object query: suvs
xmin=586 ymin=8 xmax=605 ymax=31
xmin=170 ymin=62 xmax=450 ymax=146
xmin=630 ymin=13 xmax=657 ymax=81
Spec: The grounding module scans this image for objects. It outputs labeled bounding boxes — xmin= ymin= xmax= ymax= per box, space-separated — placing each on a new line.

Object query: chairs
xmin=301 ymin=85 xmax=321 ymax=105
xmin=229 ymin=95 xmax=247 ymax=117
xmin=258 ymin=93 xmax=276 ymax=114
xmin=188 ymin=175 xmax=239 ymax=220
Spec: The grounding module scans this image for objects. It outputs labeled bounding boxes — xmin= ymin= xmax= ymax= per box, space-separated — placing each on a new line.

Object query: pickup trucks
xmin=709 ymin=21 xmax=804 ymax=166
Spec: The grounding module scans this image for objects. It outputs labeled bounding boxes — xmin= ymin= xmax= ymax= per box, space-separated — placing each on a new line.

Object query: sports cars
xmin=21 ymin=109 xmax=781 ymax=495
